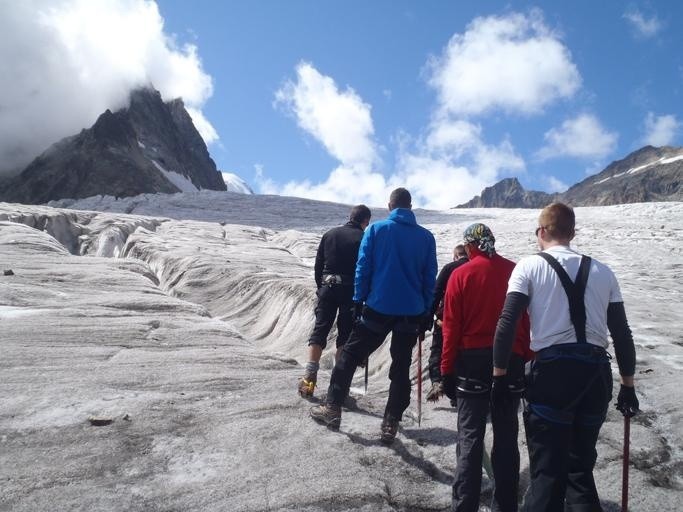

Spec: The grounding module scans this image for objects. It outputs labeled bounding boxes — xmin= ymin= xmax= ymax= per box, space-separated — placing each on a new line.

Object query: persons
xmin=492 ymin=202 xmax=639 ymax=512
xmin=298 ymin=205 xmax=371 ymax=408
xmin=426 ymin=245 xmax=470 ymax=408
xmin=309 ymin=188 xmax=436 ymax=445
xmin=441 ymin=223 xmax=532 ymax=512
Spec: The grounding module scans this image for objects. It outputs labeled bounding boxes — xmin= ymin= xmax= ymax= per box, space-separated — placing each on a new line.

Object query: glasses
xmin=536 ymin=227 xmax=547 ymax=237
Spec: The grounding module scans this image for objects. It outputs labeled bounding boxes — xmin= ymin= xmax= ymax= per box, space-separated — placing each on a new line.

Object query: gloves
xmin=488 ymin=376 xmax=509 ymax=409
xmin=614 ymin=383 xmax=639 ymax=417
xmin=349 ymin=302 xmax=363 ymax=333
xmin=442 ymin=375 xmax=456 ymax=400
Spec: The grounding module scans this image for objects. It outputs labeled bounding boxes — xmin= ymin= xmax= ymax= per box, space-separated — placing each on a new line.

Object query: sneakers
xmin=297 ymin=373 xmax=316 ymax=397
xmin=343 ymin=395 xmax=357 ymax=409
xmin=309 ymin=401 xmax=341 ymax=432
xmin=380 ymin=414 xmax=398 ymax=444
xmin=425 ymin=381 xmax=445 ymax=401
